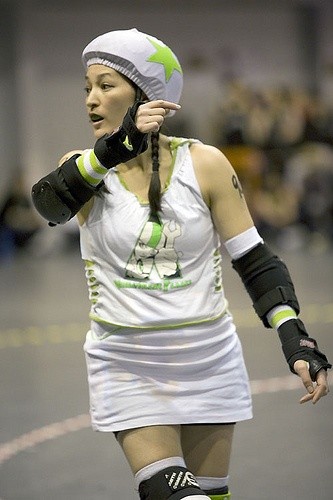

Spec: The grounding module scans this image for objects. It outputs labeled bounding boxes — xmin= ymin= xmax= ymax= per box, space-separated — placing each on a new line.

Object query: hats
xmin=81 ymin=28 xmax=183 ymax=118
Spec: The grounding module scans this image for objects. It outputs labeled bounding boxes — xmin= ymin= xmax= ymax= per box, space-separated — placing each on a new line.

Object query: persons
xmin=30 ymin=28 xmax=333 ymax=500
